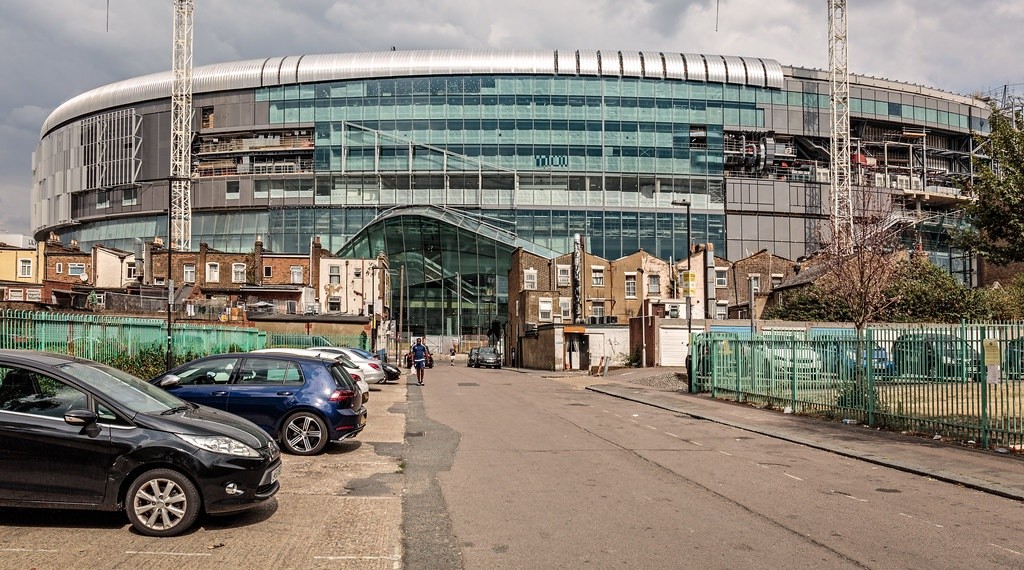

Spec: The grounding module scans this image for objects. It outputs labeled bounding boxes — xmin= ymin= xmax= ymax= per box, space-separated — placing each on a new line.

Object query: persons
xmin=450 ymin=346 xmax=456 ymax=365
xmin=411 ymin=338 xmax=430 ymax=387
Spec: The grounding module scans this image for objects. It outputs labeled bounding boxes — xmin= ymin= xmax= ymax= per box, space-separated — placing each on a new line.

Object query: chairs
xmin=253 ymin=361 xmax=267 ymax=381
xmin=242 ymin=365 xmax=252 ymax=375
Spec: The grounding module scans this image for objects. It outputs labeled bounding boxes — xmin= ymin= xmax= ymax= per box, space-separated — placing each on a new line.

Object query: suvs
xmin=150 ymin=352 xmax=367 ymax=455
xmin=686 ymin=331 xmax=822 ymax=382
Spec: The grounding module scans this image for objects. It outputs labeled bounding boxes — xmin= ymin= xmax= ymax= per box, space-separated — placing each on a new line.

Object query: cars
xmin=0 ymin=348 xmax=282 ymax=536
xmin=251 ymin=346 xmax=402 ymax=405
xmin=1005 ymin=335 xmax=1024 ymax=380
xmin=466 ymin=346 xmax=503 ymax=369
xmin=811 ymin=328 xmax=898 ymax=376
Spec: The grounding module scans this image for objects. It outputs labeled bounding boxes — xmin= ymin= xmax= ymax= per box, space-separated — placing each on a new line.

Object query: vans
xmin=890 ymin=333 xmax=980 ymax=383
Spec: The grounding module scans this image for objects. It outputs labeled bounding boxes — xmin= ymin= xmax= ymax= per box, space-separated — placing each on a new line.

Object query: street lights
xmin=671 ymin=199 xmax=692 ymax=333
xmin=135 ymin=177 xmax=192 ymax=372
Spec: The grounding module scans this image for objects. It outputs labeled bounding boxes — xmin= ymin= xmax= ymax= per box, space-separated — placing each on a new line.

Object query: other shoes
xmin=418 ymin=382 xmax=421 ymax=386
xmin=410 ymin=365 xmax=416 ymax=375
xmin=421 ymin=381 xmax=424 ymax=386
